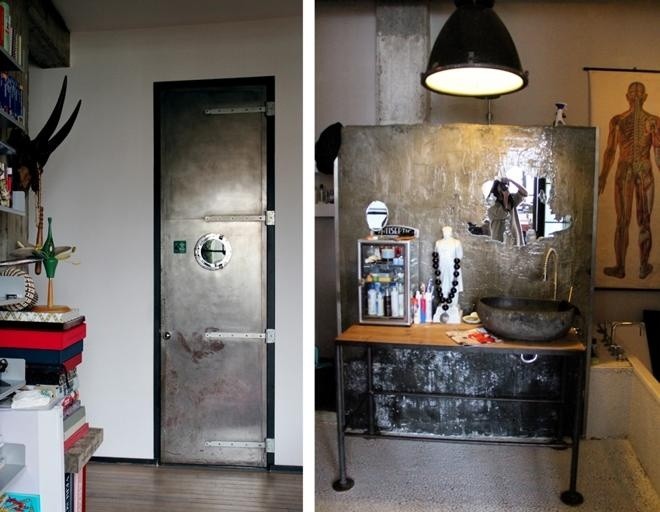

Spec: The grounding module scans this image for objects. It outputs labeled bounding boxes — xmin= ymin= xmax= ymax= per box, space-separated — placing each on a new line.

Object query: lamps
xmin=419 ymin=0 xmax=529 ymax=99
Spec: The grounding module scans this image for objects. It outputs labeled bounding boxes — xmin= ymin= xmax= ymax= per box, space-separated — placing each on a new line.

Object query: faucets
xmin=542 ymin=248 xmax=560 ymax=300
xmin=609 ymin=320 xmax=647 ymax=347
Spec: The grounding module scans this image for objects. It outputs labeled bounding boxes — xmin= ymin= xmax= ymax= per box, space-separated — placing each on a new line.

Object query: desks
xmin=329 ymin=320 xmax=590 ymax=507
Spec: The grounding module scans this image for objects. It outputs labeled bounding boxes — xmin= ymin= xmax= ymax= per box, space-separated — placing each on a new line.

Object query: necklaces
xmin=431 ymin=242 xmax=461 ymax=323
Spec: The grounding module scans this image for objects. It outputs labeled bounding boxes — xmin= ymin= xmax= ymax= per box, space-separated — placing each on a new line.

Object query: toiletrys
xmin=315 ymin=183 xmax=335 ymax=205
xmin=364 ymin=246 xmax=433 ymax=324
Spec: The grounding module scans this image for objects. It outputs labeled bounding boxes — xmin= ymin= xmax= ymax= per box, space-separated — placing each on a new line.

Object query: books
xmin=0 ymin=1 xmax=29 ymax=214
xmin=0 ymin=311 xmax=104 ymax=512
xmin=445 ymin=328 xmax=504 ymax=345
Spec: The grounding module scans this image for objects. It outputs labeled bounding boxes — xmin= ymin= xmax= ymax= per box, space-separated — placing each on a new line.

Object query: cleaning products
xmin=367 ymin=283 xmax=405 ymax=318
xmin=410 ymin=291 xmax=427 ymax=324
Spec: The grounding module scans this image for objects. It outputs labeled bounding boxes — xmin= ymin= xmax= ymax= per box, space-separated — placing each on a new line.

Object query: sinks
xmin=477 ymin=294 xmax=576 ymax=340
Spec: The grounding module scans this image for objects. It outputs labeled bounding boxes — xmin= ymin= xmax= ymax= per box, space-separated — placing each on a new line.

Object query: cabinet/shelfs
xmin=0 ymin=3 xmax=31 ymax=276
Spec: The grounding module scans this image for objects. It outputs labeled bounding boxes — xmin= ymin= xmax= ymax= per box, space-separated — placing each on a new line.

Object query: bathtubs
xmin=599 ymin=316 xmax=660 ymax=406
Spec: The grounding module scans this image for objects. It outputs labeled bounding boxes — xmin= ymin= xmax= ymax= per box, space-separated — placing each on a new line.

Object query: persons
xmin=597 ymin=81 xmax=660 ymax=280
xmin=485 ymin=177 xmax=528 ymax=246
xmin=431 ymin=226 xmax=464 ymax=306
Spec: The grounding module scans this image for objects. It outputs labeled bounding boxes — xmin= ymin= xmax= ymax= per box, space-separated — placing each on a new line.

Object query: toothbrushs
xmin=427 ymin=278 xmax=434 ymax=295
